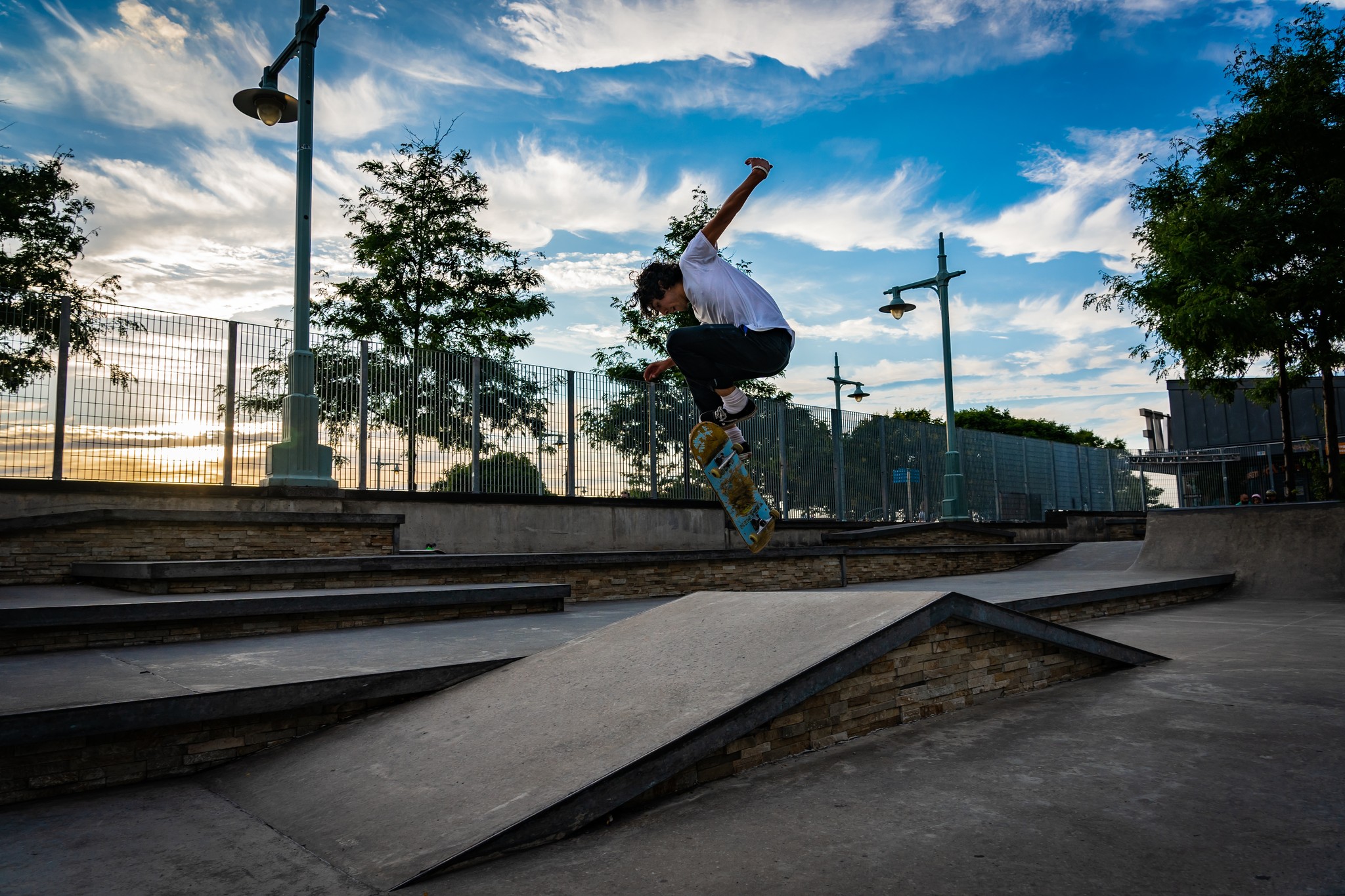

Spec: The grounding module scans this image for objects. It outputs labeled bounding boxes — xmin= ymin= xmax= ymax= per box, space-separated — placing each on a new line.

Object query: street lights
xmin=232 ymin=0 xmax=342 ymax=491
xmin=536 ymin=426 xmax=568 ymax=495
xmin=878 ymin=235 xmax=978 ymax=520
xmin=370 ymin=447 xmax=405 ymax=490
xmin=827 ymin=351 xmax=871 ymax=520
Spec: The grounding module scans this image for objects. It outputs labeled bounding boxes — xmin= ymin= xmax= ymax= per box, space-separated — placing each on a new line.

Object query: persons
xmin=632 ymin=157 xmax=796 ymax=461
xmin=1236 ymin=489 xmax=1276 ymax=506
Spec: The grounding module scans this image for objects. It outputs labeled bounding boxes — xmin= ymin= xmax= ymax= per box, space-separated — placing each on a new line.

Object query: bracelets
xmin=752 ymin=165 xmax=768 ymax=180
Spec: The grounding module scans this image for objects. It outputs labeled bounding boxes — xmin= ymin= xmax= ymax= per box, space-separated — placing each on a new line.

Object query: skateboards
xmin=689 ymin=421 xmax=780 ymax=553
xmin=399 ymin=542 xmax=447 ymax=555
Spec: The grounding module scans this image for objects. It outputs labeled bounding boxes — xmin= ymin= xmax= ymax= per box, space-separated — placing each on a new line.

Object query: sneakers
xmin=735 ymin=441 xmax=752 ymax=460
xmin=698 ymin=397 xmax=757 ymax=430
